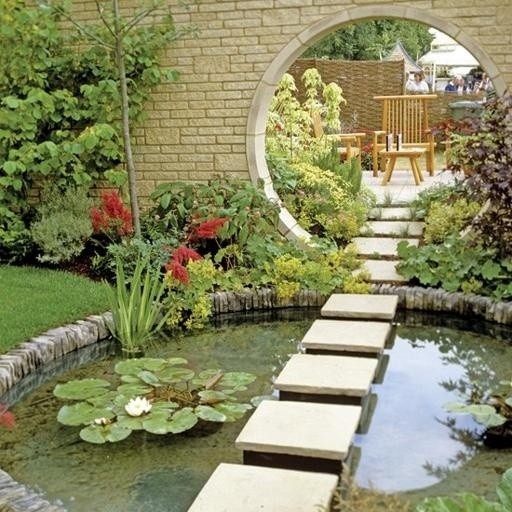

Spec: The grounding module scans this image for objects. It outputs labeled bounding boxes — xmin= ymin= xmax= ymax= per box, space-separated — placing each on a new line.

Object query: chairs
xmin=373 ymin=94 xmax=437 ymax=178
xmin=309 ymin=111 xmax=366 ymax=173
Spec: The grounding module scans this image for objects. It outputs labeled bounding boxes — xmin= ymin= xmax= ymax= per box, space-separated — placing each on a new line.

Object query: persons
xmin=407 ymin=72 xmax=430 ymax=93
xmin=455 ymin=77 xmax=467 ymax=92
xmin=444 ymin=80 xmax=458 ymax=91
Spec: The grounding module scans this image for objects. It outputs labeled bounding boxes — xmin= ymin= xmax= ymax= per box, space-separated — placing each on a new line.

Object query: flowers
xmin=124 ymin=397 xmax=153 ymax=418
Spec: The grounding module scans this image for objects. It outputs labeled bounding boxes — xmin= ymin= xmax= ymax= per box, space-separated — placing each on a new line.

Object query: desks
xmin=379 ymin=148 xmax=427 ymax=185
